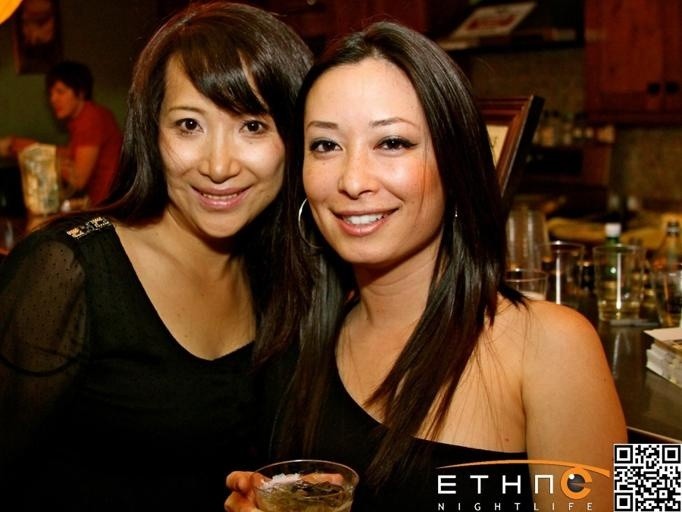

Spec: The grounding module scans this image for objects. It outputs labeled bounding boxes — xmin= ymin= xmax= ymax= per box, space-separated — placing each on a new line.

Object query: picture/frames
xmin=470 ymin=94 xmax=545 ymax=212
xmin=10 ymin=0 xmax=63 ymax=75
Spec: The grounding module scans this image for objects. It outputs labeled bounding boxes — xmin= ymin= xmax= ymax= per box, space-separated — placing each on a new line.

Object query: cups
xmin=14 ymin=154 xmax=75 ymax=218
xmin=503 ymin=266 xmax=549 ymax=301
xmin=503 ymin=208 xmax=550 ymax=270
xmin=593 ymin=242 xmax=645 ymax=321
xmin=534 ymin=237 xmax=583 ymax=309
xmin=599 ymin=328 xmax=644 ymax=407
xmin=649 ymin=268 xmax=681 ymax=327
xmin=249 ymin=457 xmax=361 ymax=512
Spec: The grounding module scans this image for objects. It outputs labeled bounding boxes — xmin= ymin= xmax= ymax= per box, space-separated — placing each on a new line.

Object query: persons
xmin=2 ymin=56 xmax=122 ymax=209
xmin=3 ymin=1 xmax=349 ymax=512
xmin=224 ymin=22 xmax=628 ymax=511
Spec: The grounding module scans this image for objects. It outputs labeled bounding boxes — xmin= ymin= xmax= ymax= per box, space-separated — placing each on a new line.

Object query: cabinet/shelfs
xmin=584 ymin=0 xmax=682 ymax=128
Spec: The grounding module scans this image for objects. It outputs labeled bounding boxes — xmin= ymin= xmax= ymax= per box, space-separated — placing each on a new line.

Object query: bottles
xmin=651 ymin=217 xmax=680 ymax=268
xmin=600 ymin=222 xmax=622 ymax=280
